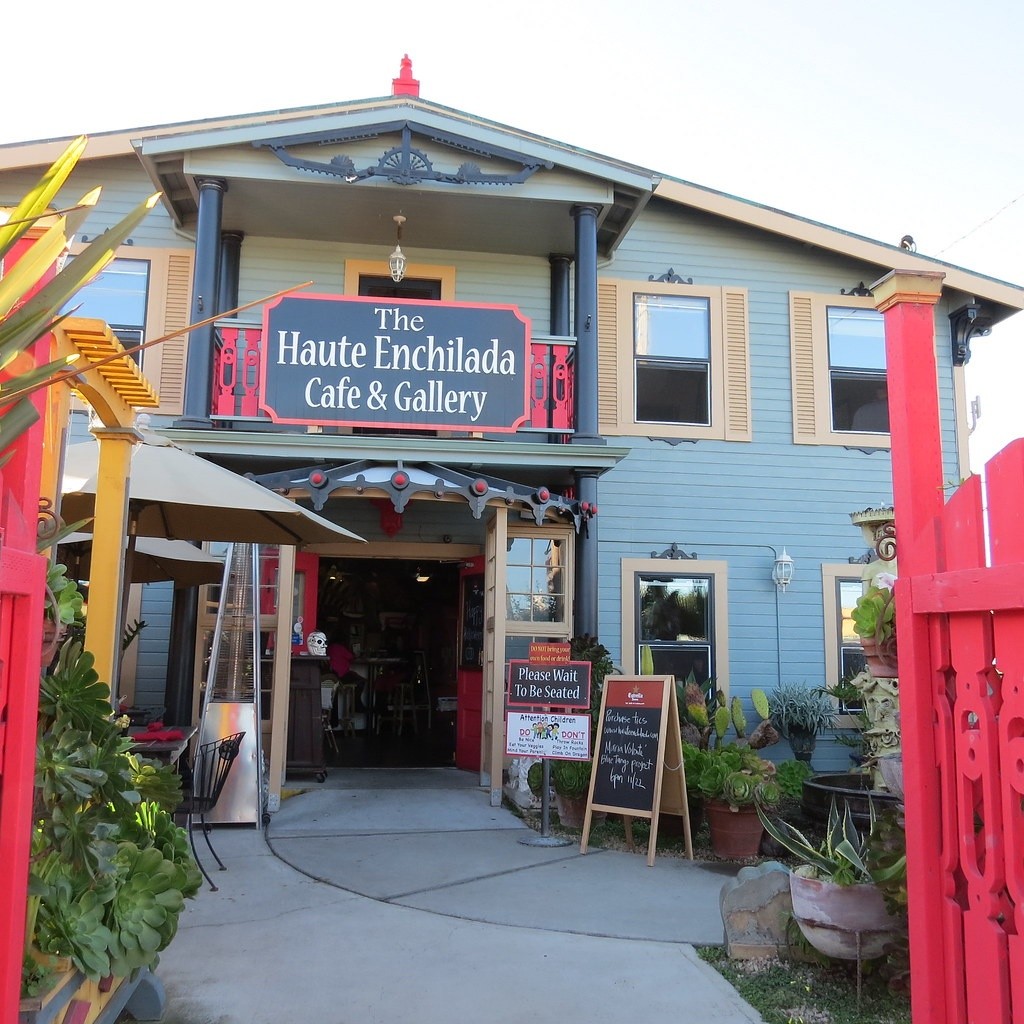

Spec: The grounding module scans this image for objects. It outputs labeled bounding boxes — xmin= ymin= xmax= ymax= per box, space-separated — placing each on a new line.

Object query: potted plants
xmin=746 ymin=787 xmax=908 ymax=961
xmin=525 ymin=758 xmax=608 ymax=829
xmin=850 ymin=584 xmax=899 ymax=679
xmin=657 ymin=646 xmax=780 ymax=836
xmin=682 ymin=741 xmax=781 ymax=861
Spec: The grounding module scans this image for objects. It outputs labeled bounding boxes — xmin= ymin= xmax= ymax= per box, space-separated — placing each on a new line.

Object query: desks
xmin=111 ymin=724 xmax=198 ymax=825
xmin=349 ymin=657 xmax=402 ymax=740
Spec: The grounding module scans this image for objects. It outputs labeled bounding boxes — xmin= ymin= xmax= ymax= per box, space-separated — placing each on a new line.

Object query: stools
xmin=389 ymin=682 xmax=417 ymax=736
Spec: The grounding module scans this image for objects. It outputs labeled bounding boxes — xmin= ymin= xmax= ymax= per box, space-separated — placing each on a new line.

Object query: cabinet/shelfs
xmin=206 ymin=656 xmax=328 ymax=782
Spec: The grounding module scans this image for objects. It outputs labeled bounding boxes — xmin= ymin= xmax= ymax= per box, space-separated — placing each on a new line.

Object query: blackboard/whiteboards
xmin=586 ymin=674 xmax=689 ymax=818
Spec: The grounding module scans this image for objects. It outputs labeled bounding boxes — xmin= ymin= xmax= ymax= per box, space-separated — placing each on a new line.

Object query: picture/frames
xmin=508 ymin=659 xmax=591 ymax=709
xmin=504 ymin=709 xmax=591 ymax=761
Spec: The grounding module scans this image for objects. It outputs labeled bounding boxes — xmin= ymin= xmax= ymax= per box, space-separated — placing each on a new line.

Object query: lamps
xmin=389 ymin=216 xmax=409 ymax=284
xmin=415 ymin=564 xmax=430 ymax=582
xmin=772 ymin=545 xmax=795 ymax=595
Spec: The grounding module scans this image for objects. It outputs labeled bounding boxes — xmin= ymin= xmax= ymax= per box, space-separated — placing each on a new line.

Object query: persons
xmin=371 ymin=634 xmax=416 ymax=717
xmin=328 ymin=634 xmax=367 ymax=714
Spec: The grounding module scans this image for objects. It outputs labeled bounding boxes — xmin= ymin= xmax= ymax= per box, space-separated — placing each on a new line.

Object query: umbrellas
xmin=56 ymin=532 xmax=225 ymax=585
xmin=59 ymin=414 xmax=369 ymax=699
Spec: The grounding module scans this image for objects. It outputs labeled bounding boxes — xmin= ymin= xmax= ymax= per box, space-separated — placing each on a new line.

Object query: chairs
xmin=321 ymin=680 xmax=342 ymax=754
xmin=168 ymin=731 xmax=247 ymax=891
